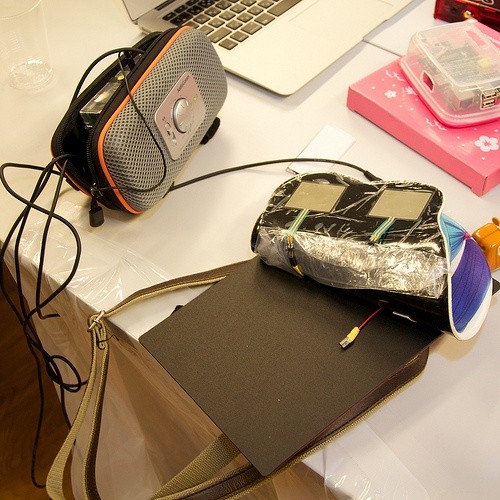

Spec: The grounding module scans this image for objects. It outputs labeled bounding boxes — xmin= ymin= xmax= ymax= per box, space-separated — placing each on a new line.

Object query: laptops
xmin=123 ymin=0 xmax=414 ymax=96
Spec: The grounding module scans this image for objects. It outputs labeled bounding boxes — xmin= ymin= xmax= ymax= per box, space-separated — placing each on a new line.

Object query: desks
xmin=0 ymin=0 xmax=500 ymax=500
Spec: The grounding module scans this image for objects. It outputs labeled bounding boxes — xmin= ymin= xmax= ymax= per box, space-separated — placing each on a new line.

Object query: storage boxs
xmin=346 ymin=20 xmax=500 ymax=196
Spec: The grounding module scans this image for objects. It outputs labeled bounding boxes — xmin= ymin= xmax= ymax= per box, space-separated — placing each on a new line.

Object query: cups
xmin=0 ymin=0 xmax=56 ymax=92
